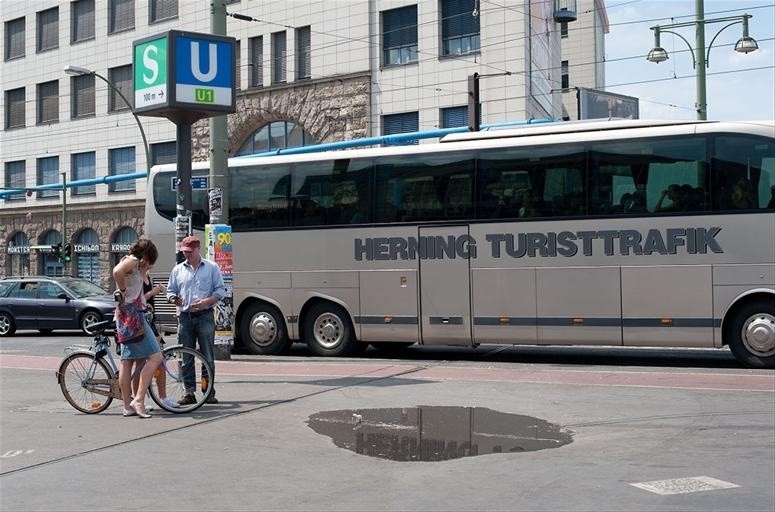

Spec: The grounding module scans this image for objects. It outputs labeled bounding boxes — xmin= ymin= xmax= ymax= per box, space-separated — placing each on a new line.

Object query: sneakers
xmin=177 ymin=393 xmax=198 ymax=405
xmin=205 ymin=397 xmax=219 ymax=405
xmin=143 ymin=397 xmax=181 ymax=411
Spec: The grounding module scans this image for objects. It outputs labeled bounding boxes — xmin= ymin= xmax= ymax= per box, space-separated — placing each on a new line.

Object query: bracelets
xmin=120 ymin=287 xmax=127 ymax=293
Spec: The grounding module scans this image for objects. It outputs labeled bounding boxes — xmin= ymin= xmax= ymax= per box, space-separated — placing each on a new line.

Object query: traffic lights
xmin=51 ymin=242 xmax=75 ymax=262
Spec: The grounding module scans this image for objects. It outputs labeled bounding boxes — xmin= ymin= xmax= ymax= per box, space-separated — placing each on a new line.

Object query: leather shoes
xmin=129 ymin=398 xmax=152 ymax=419
xmin=122 ymin=407 xmax=136 ymax=416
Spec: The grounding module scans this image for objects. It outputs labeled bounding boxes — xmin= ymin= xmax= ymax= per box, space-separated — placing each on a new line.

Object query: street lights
xmin=646 ymin=0 xmax=759 ymax=120
xmin=64 ymin=65 xmax=151 ymax=184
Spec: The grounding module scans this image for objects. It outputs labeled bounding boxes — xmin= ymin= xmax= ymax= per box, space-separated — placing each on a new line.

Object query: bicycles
xmin=56 ymin=319 xmax=214 ymax=414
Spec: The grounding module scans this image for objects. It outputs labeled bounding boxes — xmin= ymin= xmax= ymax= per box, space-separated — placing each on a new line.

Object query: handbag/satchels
xmin=115 ymin=288 xmax=146 ymax=345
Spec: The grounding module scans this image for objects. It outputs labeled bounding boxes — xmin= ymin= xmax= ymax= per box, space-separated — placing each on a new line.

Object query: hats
xmin=179 ymin=235 xmax=201 ymax=253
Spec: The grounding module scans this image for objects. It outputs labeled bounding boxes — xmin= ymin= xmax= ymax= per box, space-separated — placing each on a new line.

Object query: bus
xmin=144 ymin=118 xmax=775 ymax=369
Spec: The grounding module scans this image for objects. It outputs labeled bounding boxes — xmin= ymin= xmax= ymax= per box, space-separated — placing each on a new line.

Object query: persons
xmin=166 ymin=236 xmax=226 ymax=406
xmin=112 ymin=237 xmax=164 ymax=418
xmin=132 ymin=262 xmax=181 ymax=413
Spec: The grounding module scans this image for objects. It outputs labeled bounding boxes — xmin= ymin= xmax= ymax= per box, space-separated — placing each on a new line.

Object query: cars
xmin=0 ymin=274 xmax=116 ymax=336
